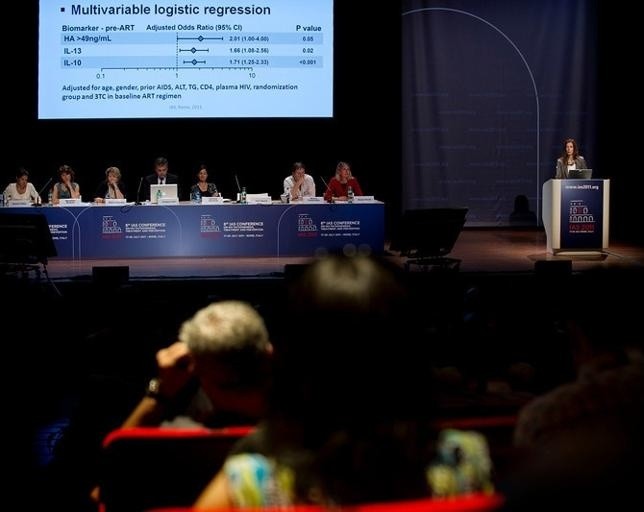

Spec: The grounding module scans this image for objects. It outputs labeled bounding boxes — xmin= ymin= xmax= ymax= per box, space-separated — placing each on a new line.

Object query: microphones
xmin=31 ymin=177 xmax=54 ymax=207
xmin=134 ymin=177 xmax=143 ymax=206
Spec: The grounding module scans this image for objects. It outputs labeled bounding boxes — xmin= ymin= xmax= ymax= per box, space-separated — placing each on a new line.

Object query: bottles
xmin=348 ymin=187 xmax=352 ymax=204
xmin=286 ymin=187 xmax=290 ymax=203
xmin=48 ymin=192 xmax=53 ymax=206
xmin=106 ymin=194 xmax=110 ymax=198
xmin=241 ymin=187 xmax=246 ymax=204
xmin=3 ymin=191 xmax=7 ymax=204
xmin=157 ymin=190 xmax=161 ymax=199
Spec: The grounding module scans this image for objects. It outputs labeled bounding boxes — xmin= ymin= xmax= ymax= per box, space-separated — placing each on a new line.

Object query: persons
xmin=91 ymin=254 xmax=644 ymax=509
xmin=143 ymin=157 xmax=183 ymax=201
xmin=94 ymin=167 xmax=128 ymax=203
xmin=555 ymin=138 xmax=588 ymax=179
xmin=324 ymin=161 xmax=363 ymax=203
xmin=3 ymin=169 xmax=42 ymax=204
xmin=190 ymin=165 xmax=219 ymax=200
xmin=52 ymin=165 xmax=80 ymax=204
xmin=284 ymin=162 xmax=316 ymax=201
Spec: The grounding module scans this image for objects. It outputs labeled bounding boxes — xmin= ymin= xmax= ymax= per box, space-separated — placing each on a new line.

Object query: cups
xmin=297 ymin=191 xmax=303 ymax=201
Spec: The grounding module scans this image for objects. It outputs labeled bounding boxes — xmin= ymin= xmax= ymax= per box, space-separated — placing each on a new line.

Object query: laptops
xmin=567 ymin=168 xmax=594 ymax=179
xmin=150 ymin=183 xmax=178 ymax=204
